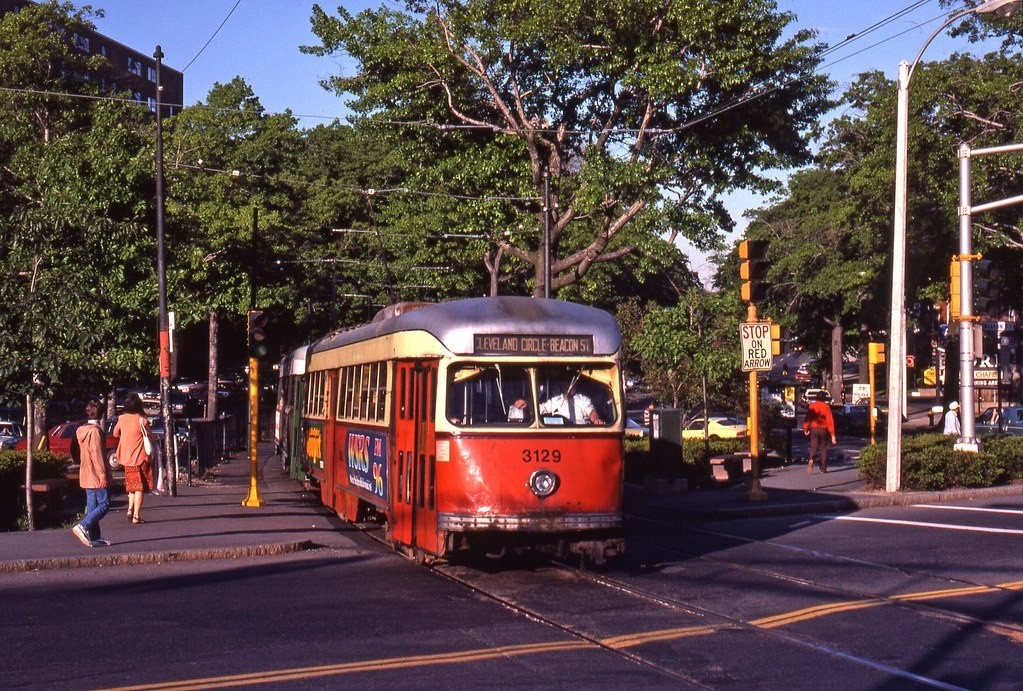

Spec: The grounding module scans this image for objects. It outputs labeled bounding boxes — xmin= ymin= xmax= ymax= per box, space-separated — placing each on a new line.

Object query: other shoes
xmin=821 ymin=470 xmax=829 ymax=474
xmin=808 ymin=461 xmax=814 ymax=474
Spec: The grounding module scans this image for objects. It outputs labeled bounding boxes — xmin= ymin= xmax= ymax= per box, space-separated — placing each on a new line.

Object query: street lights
xmin=884 ymin=0 xmax=1023 ymax=493
xmin=153 ymin=45 xmax=177 ymax=497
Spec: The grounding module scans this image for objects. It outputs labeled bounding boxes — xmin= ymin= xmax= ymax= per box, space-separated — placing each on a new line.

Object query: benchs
xmin=710 ymin=453 xmax=747 ymax=482
xmin=19 ymin=479 xmax=66 ymax=508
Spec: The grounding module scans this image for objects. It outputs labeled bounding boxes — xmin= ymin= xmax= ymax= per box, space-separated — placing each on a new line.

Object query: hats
xmin=949 ymin=401 xmax=960 ymax=410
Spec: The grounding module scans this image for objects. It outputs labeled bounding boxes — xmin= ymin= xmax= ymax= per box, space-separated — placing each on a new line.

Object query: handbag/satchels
xmin=139 ymin=416 xmax=154 ymax=456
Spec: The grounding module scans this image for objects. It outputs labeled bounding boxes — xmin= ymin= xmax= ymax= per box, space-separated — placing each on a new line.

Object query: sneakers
xmin=90 ymin=538 xmax=115 ymax=547
xmin=73 ymin=523 xmax=95 ymax=548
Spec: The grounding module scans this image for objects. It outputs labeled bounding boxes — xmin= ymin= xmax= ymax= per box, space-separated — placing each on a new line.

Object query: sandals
xmin=127 ymin=509 xmax=134 ymax=522
xmin=133 ymin=518 xmax=145 ymax=525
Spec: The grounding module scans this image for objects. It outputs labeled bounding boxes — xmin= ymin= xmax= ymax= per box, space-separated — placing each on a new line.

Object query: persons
xmin=803 ymin=391 xmax=837 ymax=473
xmin=991 ymin=408 xmax=1004 ymax=425
xmin=943 ymin=401 xmax=961 ymax=436
xmin=644 ymin=403 xmax=654 ymax=427
xmin=113 ymin=394 xmax=156 ymax=524
xmin=72 ymin=398 xmax=111 ymax=546
xmin=514 ymin=372 xmax=608 ymax=425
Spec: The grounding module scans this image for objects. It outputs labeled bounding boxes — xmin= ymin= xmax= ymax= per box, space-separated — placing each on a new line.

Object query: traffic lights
xmin=868 ymin=342 xmax=886 ymax=364
xmin=738 ymin=238 xmax=773 ymax=302
xmin=966 ymin=255 xmax=993 ymax=319
xmin=246 ymin=310 xmax=271 ymax=360
xmin=770 ymin=324 xmax=790 ymax=356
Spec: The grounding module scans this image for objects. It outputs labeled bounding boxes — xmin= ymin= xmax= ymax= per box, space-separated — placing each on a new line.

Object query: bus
xmin=272 ymin=295 xmax=626 ymax=566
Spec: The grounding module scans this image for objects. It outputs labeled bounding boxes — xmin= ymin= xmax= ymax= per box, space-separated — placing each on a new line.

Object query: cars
xmin=831 ymin=404 xmax=888 ymax=433
xmin=0 ymin=364 xmax=281 ymax=471
xmin=974 ymin=406 xmax=1023 ymax=436
xmin=680 ymin=417 xmax=762 ymax=442
xmin=800 ymin=388 xmax=835 ymax=408
xmin=626 ymin=378 xmax=643 ymax=389
xmin=795 ymin=356 xmax=819 ymax=385
